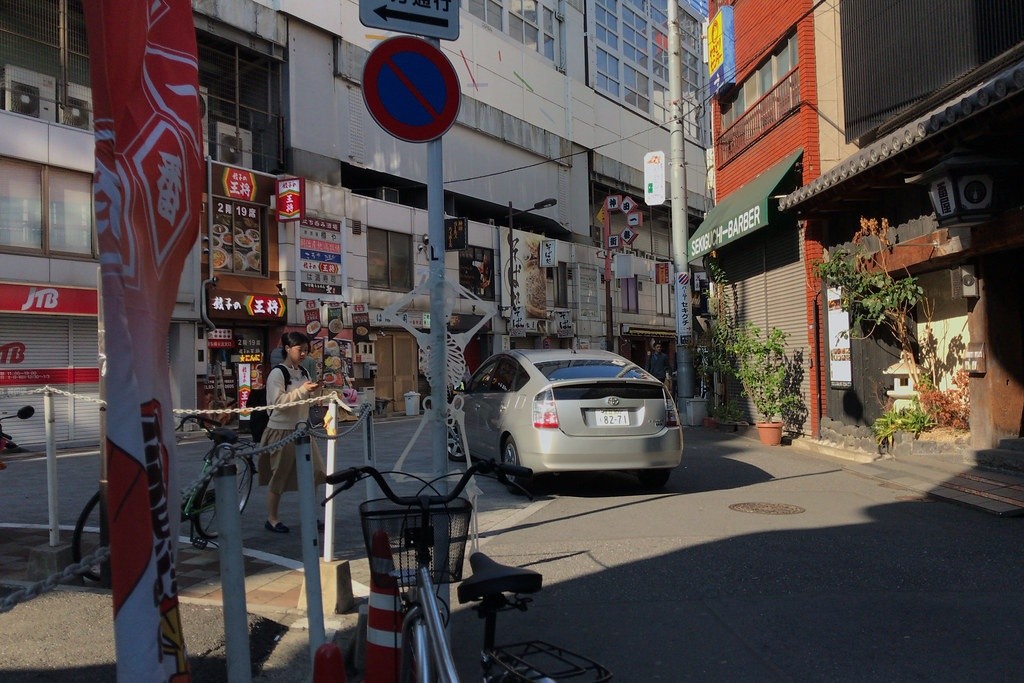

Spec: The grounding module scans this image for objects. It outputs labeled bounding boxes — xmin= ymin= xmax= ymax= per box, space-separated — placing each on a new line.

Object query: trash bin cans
xmin=403 ymin=391 xmax=421 ymax=416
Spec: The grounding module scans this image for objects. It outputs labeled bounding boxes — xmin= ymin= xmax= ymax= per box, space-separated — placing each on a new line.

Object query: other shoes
xmin=316 ymin=520 xmax=324 ymax=532
xmin=265 ymin=520 xmax=289 ymax=533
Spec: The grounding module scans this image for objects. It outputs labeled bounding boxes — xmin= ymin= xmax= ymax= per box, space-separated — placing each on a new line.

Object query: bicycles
xmin=72 ymin=414 xmax=261 ymax=583
xmin=315 ymin=456 xmax=615 ymax=683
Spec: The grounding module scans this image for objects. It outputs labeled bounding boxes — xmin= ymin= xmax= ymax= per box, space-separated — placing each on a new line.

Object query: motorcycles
xmin=0 ymin=405 xmax=35 ymax=471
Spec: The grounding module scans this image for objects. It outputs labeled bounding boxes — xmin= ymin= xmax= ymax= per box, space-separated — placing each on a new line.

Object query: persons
xmin=645 ymin=343 xmax=666 ymax=381
xmin=258 ymin=331 xmax=328 ymax=532
xmin=666 ymin=349 xmax=677 ymax=380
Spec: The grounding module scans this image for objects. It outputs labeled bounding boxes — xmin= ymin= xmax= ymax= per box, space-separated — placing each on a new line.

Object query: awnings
xmin=686 ymin=148 xmax=803 ymax=262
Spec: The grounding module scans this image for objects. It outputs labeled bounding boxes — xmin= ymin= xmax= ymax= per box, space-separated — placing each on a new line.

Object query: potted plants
xmin=722 ymin=322 xmax=797 ymax=447
xmin=716 ymin=400 xmax=744 ymax=433
xmin=703 ymin=391 xmax=716 ymax=428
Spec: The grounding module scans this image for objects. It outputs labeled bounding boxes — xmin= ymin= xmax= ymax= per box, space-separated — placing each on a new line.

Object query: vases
xmin=736 ymin=421 xmax=749 ymax=433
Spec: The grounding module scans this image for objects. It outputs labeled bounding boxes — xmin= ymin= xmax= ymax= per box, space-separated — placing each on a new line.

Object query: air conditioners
xmin=56 ymin=80 xmax=94 ymax=132
xmin=199 ymin=84 xmax=209 ymax=157
xmin=374 ymin=186 xmax=399 ymax=204
xmin=0 ymin=64 xmax=56 ymax=123
xmin=208 ymin=121 xmax=252 ymax=170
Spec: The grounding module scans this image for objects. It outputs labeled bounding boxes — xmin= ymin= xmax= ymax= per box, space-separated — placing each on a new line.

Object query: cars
xmin=447 ymin=347 xmax=684 ymax=497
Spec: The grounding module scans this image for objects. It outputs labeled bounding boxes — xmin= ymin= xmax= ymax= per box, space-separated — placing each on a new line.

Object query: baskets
xmin=359 ymin=495 xmax=473 ymax=589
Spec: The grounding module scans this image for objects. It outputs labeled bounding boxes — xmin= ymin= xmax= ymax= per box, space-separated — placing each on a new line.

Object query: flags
xmin=86 ymin=0 xmax=203 ymax=683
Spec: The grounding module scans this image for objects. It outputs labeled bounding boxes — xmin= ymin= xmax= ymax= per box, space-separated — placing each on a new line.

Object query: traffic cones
xmin=314 ymin=643 xmax=349 ymax=683
xmin=363 ymin=525 xmax=409 ymax=683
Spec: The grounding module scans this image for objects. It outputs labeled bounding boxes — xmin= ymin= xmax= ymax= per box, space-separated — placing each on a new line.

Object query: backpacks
xmin=246 ymin=365 xmax=308 ymax=443
